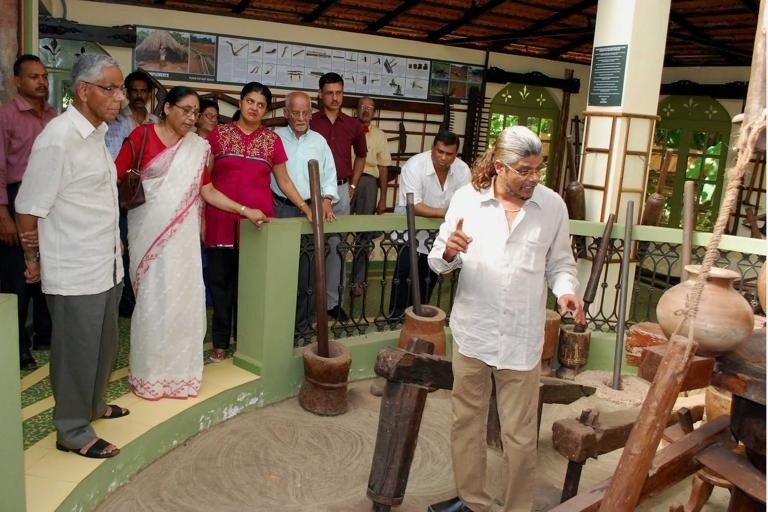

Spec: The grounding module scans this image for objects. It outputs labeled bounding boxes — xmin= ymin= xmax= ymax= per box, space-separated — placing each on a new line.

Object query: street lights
xmin=118 ymin=124 xmax=148 ymax=210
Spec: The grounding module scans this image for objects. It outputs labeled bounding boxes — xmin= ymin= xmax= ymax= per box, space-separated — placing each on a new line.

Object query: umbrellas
xmin=352 ymin=283 xmax=363 ymax=295
xmin=330 ymin=307 xmax=348 ymax=321
xmin=33 ymin=330 xmax=51 ymax=349
xmin=21 ymin=347 xmax=37 ymax=370
xmin=429 ymin=496 xmax=472 ymax=512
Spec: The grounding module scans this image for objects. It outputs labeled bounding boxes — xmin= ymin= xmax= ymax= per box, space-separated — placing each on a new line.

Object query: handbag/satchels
xmin=240 ymin=205 xmax=245 ymax=215
xmin=300 ymin=202 xmax=306 ymax=210
xmin=324 ymin=197 xmax=332 ymax=201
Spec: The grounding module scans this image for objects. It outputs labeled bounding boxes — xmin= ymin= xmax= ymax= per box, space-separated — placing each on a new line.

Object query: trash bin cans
xmin=351 ymin=184 xmax=355 ymax=189
xmin=24 ymin=251 xmax=40 ymax=262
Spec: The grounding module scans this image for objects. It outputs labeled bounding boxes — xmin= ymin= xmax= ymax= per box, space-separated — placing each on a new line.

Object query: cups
xmin=503 ymin=162 xmax=546 ymax=179
xmin=174 ymin=103 xmax=201 ymax=119
xmin=287 ymin=108 xmax=312 ymax=117
xmin=82 ymin=79 xmax=127 ymax=99
xmin=200 ymin=112 xmax=219 ymax=120
xmin=359 ymin=104 xmax=374 ymax=110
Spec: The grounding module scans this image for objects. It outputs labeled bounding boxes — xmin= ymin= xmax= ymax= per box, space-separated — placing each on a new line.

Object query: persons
xmin=270 ymin=93 xmax=340 ymax=347
xmin=232 ymin=110 xmax=240 ymax=122
xmin=428 ymin=126 xmax=587 ymax=512
xmin=351 ymin=97 xmax=394 ymax=294
xmin=202 ymin=82 xmax=313 ymax=363
xmin=16 ymin=52 xmax=129 ymax=459
xmin=309 ymin=72 xmax=369 ymax=320
xmin=1 ymin=54 xmax=59 ymax=372
xmin=103 ymin=71 xmax=162 ymax=316
xmin=388 ymin=132 xmax=473 ymax=324
xmin=194 ymin=100 xmax=219 ymax=139
xmin=114 ymin=86 xmax=272 ymax=401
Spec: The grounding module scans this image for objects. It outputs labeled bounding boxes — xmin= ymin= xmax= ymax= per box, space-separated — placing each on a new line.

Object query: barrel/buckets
xmin=56 ymin=438 xmax=120 ymax=457
xmin=210 ymin=350 xmax=225 ymax=361
xmin=100 ymin=404 xmax=129 ymax=418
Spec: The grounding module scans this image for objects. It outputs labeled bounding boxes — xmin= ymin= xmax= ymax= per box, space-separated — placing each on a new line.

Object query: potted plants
xmin=217 ymin=34 xmax=432 ymax=101
xmin=132 ymin=24 xmax=218 ymax=83
xmin=429 ymin=58 xmax=486 ymax=107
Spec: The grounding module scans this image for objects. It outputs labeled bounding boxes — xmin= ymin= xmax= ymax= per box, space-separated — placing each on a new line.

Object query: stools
xmin=337 ymin=177 xmax=350 ymax=186
xmin=361 ymin=173 xmax=370 ymax=177
xmin=272 ymin=193 xmax=312 ymax=206
